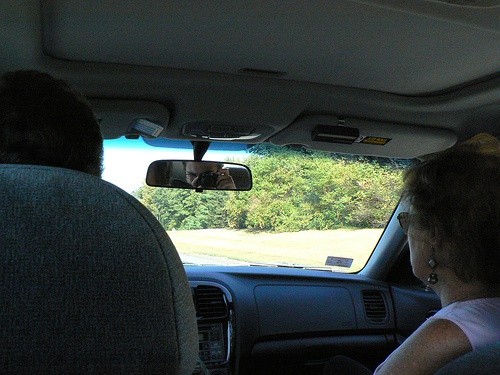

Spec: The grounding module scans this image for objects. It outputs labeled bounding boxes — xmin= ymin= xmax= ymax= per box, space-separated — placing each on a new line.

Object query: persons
xmin=323 ymin=147 xmax=500 ymax=375
xmin=1 ymin=68 xmax=210 ymax=375
xmin=147 ymin=161 xmax=173 ymax=187
xmin=183 ymin=162 xmax=237 ymax=190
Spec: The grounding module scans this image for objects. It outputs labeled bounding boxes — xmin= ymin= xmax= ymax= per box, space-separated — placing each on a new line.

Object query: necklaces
xmin=441 ymin=287 xmax=495 ymax=310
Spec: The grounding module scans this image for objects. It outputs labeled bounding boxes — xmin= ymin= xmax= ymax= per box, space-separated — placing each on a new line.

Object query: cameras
xmin=199 ymin=172 xmax=220 ymax=187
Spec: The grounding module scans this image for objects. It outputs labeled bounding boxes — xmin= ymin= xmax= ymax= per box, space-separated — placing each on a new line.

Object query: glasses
xmin=397 ymin=212 xmax=411 ymax=232
xmin=186 ymin=172 xmax=214 ymax=180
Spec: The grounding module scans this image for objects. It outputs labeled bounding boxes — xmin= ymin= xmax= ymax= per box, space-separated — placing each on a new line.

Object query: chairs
xmin=0 ymin=165 xmax=199 ymax=375
